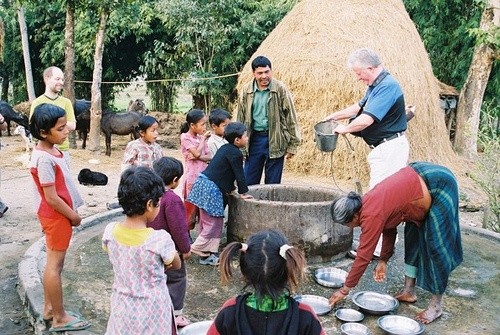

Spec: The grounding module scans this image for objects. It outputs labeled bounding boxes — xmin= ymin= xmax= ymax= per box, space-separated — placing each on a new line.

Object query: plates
xmin=311 ymin=267 xmax=349 ymax=287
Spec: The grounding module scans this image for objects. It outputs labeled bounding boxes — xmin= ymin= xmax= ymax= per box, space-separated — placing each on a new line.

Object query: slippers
xmin=199 ymin=254 xmax=220 ymax=266
xmin=176 ymin=316 xmax=191 ymax=326
xmin=49 ymin=317 xmax=91 ymax=332
xmin=348 ymin=249 xmax=379 ymax=260
xmin=43 ymin=311 xmax=79 ymax=322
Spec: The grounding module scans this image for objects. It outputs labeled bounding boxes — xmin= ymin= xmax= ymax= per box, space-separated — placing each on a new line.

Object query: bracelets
xmin=340 ymin=287 xmax=349 ymax=295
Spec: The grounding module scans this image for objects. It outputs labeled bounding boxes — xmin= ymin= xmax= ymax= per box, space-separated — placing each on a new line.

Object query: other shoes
xmin=0 ymin=207 xmax=8 ymax=218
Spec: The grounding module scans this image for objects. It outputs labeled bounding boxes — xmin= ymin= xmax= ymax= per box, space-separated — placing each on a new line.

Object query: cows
xmin=100 ymin=112 xmax=143 ymax=157
xmin=127 ymin=99 xmax=149 ymax=113
xmin=74 ymin=98 xmax=91 ymax=149
xmin=0 ymin=100 xmax=29 ymax=136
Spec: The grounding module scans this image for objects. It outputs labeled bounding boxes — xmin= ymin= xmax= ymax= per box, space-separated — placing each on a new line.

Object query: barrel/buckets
xmin=313 ymin=121 xmax=339 ymax=152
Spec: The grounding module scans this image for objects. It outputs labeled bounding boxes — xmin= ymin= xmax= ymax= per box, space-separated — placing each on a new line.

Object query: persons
xmin=29 ymin=66 xmax=76 ymax=171
xmin=101 ymin=167 xmax=181 ymax=335
xmin=122 ymin=116 xmax=165 ymax=167
xmin=187 ymin=122 xmax=255 ymax=265
xmin=0 ymin=112 xmax=8 ymax=217
xmin=318 ymin=48 xmax=410 ymax=260
xmin=146 ymin=156 xmax=192 ymax=326
xmin=206 ymin=229 xmax=327 ymax=335
xmin=236 ymin=55 xmax=302 ymax=185
xmin=328 ymin=162 xmax=464 ymax=325
xmin=179 ymin=108 xmax=213 ymax=245
xmin=28 ymin=102 xmax=92 ymax=331
xmin=206 ymin=109 xmax=234 ymax=164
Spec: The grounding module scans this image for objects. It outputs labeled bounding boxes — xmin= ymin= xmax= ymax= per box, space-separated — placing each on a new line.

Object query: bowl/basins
xmin=377 ymin=314 xmax=426 ymax=335
xmin=335 ymin=308 xmax=365 ymax=321
xmin=340 ymin=322 xmax=372 ymax=335
xmin=293 ymin=294 xmax=332 ymax=315
xmin=351 ymin=291 xmax=399 ymax=313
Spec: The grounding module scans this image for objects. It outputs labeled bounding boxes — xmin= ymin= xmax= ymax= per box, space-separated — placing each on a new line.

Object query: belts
xmin=369 ymin=132 xmax=402 ymax=149
xmin=253 ymin=131 xmax=269 ymax=135
